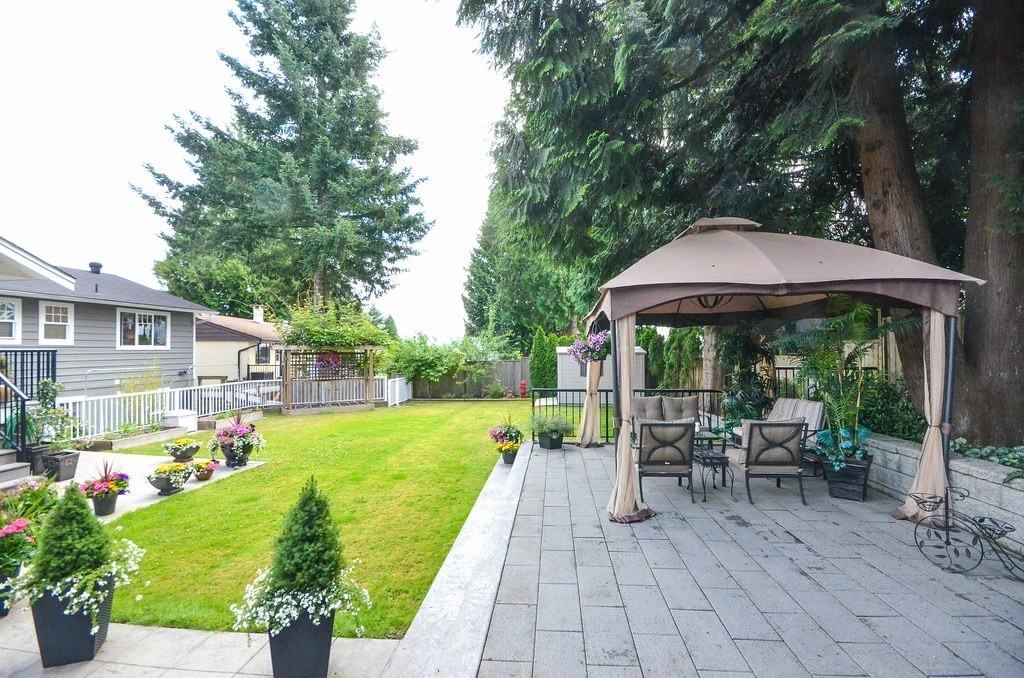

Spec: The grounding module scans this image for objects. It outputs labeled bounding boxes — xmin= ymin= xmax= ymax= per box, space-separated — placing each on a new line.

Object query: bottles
xmin=694 ymin=420 xmax=700 ymax=432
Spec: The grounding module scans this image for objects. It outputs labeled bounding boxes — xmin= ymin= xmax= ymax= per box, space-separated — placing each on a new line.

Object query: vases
xmin=502 ymin=453 xmax=516 ymax=464
xmin=269 ymin=609 xmax=334 ymax=678
xmin=221 ymin=447 xmax=253 ymax=467
xmin=0 ymin=385 xmax=11 ymax=403
xmin=92 ymin=493 xmax=118 ymax=516
xmin=167 ymin=447 xmax=199 ymax=462
xmin=0 ymin=559 xmax=22 ymax=617
xmin=594 ymin=355 xmax=606 ymax=360
xmin=11 ymin=434 xmax=80 ymax=482
xmin=195 ymin=473 xmax=213 ymax=481
xmin=28 ymin=572 xmax=115 ymax=668
xmin=147 ymin=473 xmax=192 ymax=496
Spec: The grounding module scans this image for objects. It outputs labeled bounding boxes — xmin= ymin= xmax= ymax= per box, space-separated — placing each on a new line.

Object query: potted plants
xmin=710 ymin=321 xmax=790 ymax=449
xmin=761 ymin=293 xmax=924 ymax=503
xmin=525 ymin=407 xmax=575 ymax=449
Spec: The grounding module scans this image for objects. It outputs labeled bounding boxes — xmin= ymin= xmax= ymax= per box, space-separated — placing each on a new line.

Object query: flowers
xmin=230 ymin=474 xmax=373 ymax=647
xmin=0 ymin=353 xmax=13 ymax=385
xmin=161 ymin=438 xmax=203 ymax=455
xmin=192 ymin=459 xmax=220 ymax=475
xmin=2 ymin=378 xmax=96 ymax=454
xmin=79 ymin=458 xmax=130 ymax=501
xmin=568 ymin=329 xmax=611 ymax=365
xmin=148 ymin=462 xmax=194 ymax=488
xmin=0 ymin=468 xmax=151 ymax=635
xmin=316 ymin=350 xmax=342 ymax=374
xmin=489 ymin=409 xmax=524 ymax=454
xmin=208 ymin=410 xmax=267 ymax=460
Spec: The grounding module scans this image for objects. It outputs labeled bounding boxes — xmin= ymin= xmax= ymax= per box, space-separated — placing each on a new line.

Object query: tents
xmin=575 ymin=216 xmax=984 ymax=526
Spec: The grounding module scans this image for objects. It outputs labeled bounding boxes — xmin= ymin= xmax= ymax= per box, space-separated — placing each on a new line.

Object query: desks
xmin=695 ymin=431 xmax=719 ymax=449
xmin=693 ymin=446 xmax=738 ymax=503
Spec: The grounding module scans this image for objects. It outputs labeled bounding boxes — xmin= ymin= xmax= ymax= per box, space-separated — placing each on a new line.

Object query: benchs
xmin=732 ymin=397 xmax=828 ymax=480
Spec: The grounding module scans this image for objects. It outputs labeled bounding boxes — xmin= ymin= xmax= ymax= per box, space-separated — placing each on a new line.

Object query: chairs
xmin=632 ymin=395 xmax=719 ymax=450
xmin=724 ymin=416 xmax=809 ymax=506
xmin=630 ymin=417 xmax=696 ymax=504
